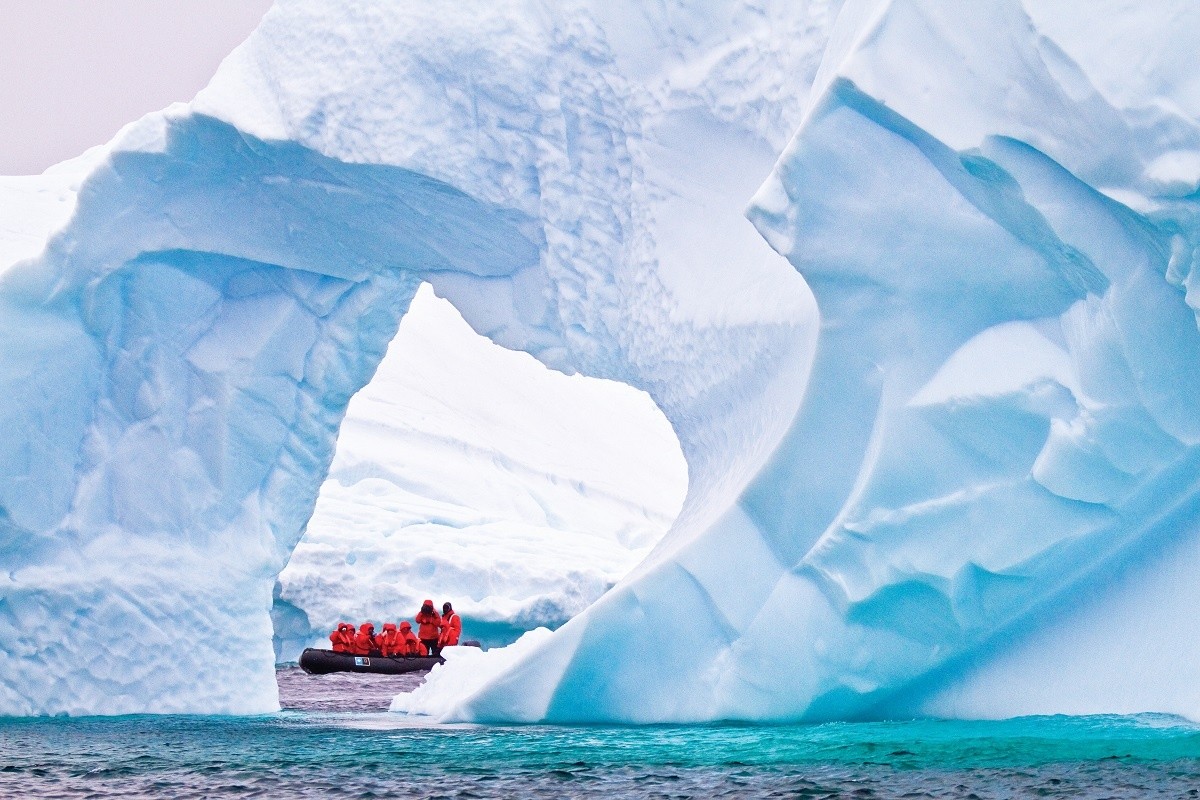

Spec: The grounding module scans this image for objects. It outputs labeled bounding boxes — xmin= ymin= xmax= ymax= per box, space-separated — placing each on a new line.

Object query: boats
xmin=298 ymin=641 xmax=482 ymax=676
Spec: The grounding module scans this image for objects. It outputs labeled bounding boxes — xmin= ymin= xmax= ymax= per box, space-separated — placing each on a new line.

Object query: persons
xmin=436 ymin=602 xmax=462 ymax=655
xmin=416 ymin=600 xmax=440 ymax=655
xmin=330 ymin=623 xmax=427 ymax=656
